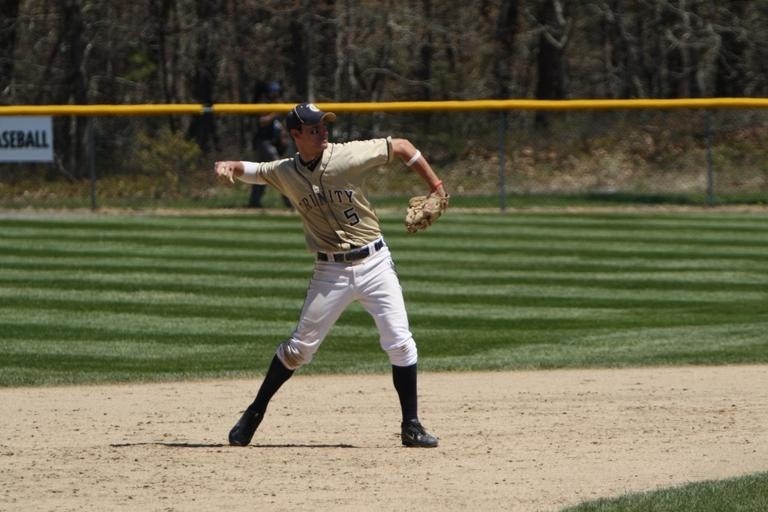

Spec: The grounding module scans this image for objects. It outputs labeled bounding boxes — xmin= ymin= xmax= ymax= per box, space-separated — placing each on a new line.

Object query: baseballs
xmin=217 ymin=168 xmax=232 ymax=185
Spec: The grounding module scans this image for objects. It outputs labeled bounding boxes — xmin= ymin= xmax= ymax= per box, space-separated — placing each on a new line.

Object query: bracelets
xmin=432 ymin=180 xmax=443 ymax=192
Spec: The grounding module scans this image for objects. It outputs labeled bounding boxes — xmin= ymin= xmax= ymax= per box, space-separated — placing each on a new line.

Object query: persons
xmin=215 ymin=104 xmax=450 ymax=447
xmin=247 ymin=80 xmax=294 ymax=208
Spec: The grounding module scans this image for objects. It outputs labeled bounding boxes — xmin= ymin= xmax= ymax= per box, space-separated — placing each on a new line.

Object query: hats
xmin=264 ymin=81 xmax=283 ymax=92
xmin=284 ymin=102 xmax=339 ymax=129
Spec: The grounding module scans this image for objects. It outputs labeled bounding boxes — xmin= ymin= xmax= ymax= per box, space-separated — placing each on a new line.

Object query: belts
xmin=316 ymin=240 xmax=385 ymax=265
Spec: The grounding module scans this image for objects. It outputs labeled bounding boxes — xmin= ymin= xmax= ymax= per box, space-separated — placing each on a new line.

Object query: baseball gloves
xmin=407 ymin=192 xmax=451 ymax=233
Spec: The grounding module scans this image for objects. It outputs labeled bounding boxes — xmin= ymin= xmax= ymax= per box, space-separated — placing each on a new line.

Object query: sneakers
xmin=398 ymin=418 xmax=438 ymax=450
xmin=228 ymin=401 xmax=265 ymax=447
